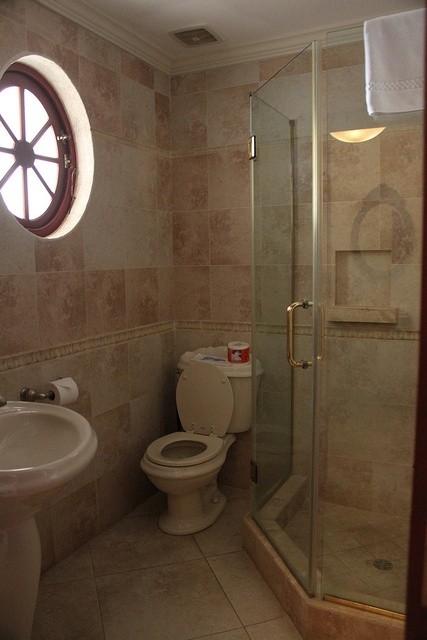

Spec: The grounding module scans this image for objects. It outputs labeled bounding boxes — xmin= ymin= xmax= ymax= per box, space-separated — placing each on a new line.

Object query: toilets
xmin=141 ymin=348 xmax=264 ymax=534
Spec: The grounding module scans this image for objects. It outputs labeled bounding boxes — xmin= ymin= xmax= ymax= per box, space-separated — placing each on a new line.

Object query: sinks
xmin=0 ymin=400 xmax=98 ymax=495
